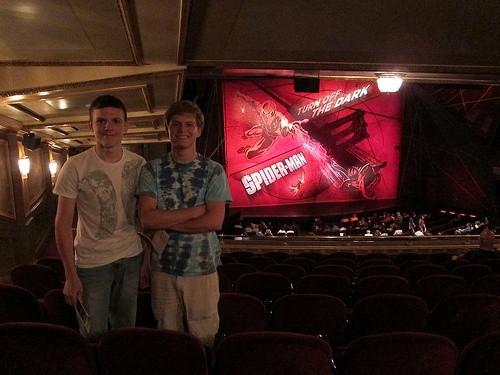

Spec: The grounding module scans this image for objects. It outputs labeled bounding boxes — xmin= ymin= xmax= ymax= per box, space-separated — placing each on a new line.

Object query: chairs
xmin=0 ymin=245 xmax=500 ymax=375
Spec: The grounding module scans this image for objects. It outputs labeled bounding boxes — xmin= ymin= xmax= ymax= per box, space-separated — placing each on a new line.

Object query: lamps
xmin=373 ymin=72 xmax=407 ymax=94
xmin=23 ymin=129 xmax=42 ymax=152
xmin=292 ymin=69 xmax=321 ymax=94
xmin=17 ymin=142 xmax=58 ymax=175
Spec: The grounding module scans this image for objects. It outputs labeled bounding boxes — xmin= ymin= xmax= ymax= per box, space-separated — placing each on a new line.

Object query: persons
xmin=133 ymin=99 xmax=231 ymax=361
xmin=455 ymin=221 xmax=494 ymax=249
xmin=245 ymin=210 xmax=427 ymax=236
xmin=51 ymin=94 xmax=146 ymax=336
